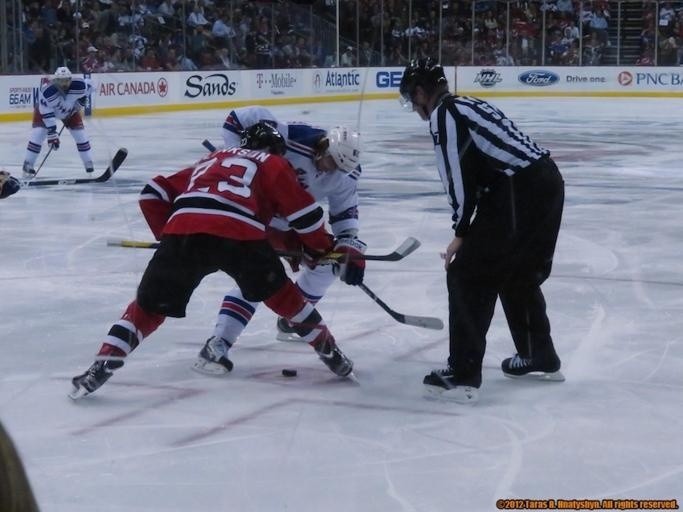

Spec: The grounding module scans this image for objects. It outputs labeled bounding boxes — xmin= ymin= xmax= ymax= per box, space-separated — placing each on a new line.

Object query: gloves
xmin=0 ymin=170 xmax=21 ymax=199
xmin=46 ymin=128 xmax=61 ymax=151
xmin=72 ymin=101 xmax=84 ymax=117
xmin=296 ymin=232 xmax=367 ymax=287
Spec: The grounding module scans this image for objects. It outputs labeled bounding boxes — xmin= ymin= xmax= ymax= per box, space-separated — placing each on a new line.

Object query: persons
xmin=398 ymin=56 xmax=565 ymax=392
xmin=198 ymin=104 xmax=369 ymax=371
xmin=22 ymin=66 xmax=93 ymax=174
xmin=71 ymin=121 xmax=354 ymax=394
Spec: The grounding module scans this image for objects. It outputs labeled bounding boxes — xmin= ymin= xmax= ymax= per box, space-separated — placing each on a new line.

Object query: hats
xmin=87 ymin=47 xmax=99 ymax=53
xmin=82 ymin=22 xmax=90 ymax=29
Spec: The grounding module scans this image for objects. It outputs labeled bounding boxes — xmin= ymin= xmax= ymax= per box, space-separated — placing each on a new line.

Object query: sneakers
xmin=500 ymin=345 xmax=563 ymax=377
xmin=422 ymin=361 xmax=485 ymax=389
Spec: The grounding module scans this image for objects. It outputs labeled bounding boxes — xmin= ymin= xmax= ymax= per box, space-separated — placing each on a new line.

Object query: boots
xmin=70 ymin=354 xmax=124 ymax=396
xmin=197 ymin=335 xmax=237 ymax=373
xmin=309 ymin=331 xmax=353 ymax=378
xmin=23 ymin=159 xmax=36 ymax=175
xmin=277 ymin=316 xmax=299 ymax=334
xmin=82 ymin=159 xmax=94 ymax=173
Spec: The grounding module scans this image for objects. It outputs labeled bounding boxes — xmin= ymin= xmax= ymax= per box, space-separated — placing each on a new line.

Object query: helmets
xmin=399 ymin=56 xmax=447 ymax=119
xmin=238 ymin=121 xmax=287 ymax=158
xmin=312 ymin=124 xmax=366 ymax=175
xmin=52 ymin=66 xmax=74 ymax=90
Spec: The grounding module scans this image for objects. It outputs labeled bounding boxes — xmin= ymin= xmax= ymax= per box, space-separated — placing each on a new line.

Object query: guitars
xmin=202 ymin=140 xmax=444 ymax=329
xmin=17 ymin=148 xmax=128 ymax=187
xmin=107 ymin=237 xmax=421 ymax=261
xmin=23 ymin=106 xmax=74 ymax=179
